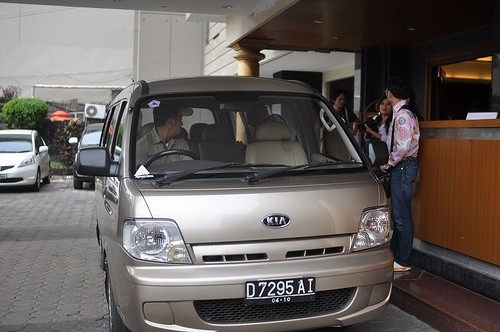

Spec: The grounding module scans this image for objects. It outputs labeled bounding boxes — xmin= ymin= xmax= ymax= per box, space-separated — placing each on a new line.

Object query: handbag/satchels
xmin=378 ymin=170 xmax=391 ymax=199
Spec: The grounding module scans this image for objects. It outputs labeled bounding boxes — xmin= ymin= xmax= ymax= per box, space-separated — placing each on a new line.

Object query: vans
xmin=68 ymin=122 xmax=104 ymax=191
xmin=73 ymin=75 xmax=396 ymax=332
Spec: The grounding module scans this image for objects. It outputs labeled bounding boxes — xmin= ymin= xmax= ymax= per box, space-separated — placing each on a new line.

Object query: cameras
xmin=356 ymin=117 xmax=377 ymax=133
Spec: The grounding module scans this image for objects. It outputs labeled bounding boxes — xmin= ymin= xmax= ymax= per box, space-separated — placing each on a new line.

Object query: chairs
xmin=244 ymin=120 xmax=309 ymax=167
xmin=138 ymin=123 xmax=187 ymax=145
xmin=188 ymin=123 xmax=237 ymax=162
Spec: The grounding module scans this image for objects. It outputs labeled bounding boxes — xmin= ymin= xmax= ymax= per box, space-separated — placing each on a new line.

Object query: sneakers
xmin=393 ymin=261 xmax=411 ymax=272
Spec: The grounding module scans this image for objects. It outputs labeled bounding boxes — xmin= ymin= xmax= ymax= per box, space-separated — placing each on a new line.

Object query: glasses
xmin=384 ymin=90 xmax=388 ymax=94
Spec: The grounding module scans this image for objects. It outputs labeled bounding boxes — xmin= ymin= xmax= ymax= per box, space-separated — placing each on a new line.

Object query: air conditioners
xmin=85 ymin=103 xmax=106 ymax=120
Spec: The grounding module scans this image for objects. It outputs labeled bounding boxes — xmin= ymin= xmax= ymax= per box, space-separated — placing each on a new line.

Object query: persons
xmin=356 ymin=97 xmax=394 ymax=217
xmin=323 ymin=89 xmax=360 ymax=136
xmin=135 ymin=101 xmax=195 ymax=170
xmin=379 ymin=74 xmax=421 ymax=272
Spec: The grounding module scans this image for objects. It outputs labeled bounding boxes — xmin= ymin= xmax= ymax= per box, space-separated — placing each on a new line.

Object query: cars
xmin=0 ymin=129 xmax=52 ymax=193
